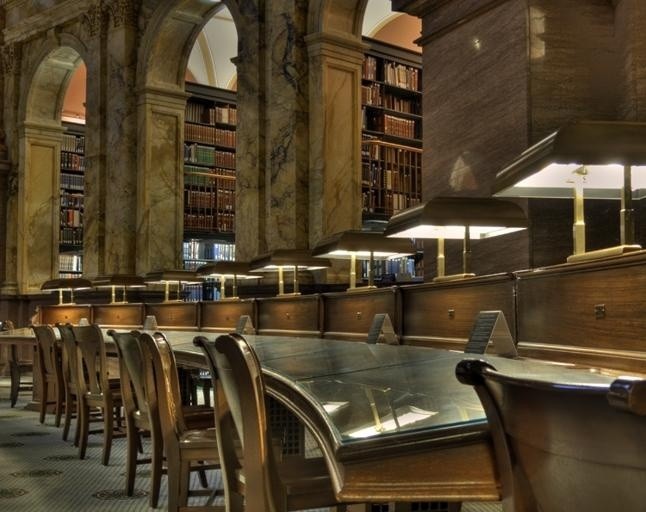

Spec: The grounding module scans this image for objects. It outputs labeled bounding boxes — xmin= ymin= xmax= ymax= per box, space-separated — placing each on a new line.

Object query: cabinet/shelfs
xmin=362 ymin=36 xmax=423 ymax=282
xmin=183 ymin=81 xmax=237 ymax=272
xmin=59 ymin=120 xmax=86 ymax=280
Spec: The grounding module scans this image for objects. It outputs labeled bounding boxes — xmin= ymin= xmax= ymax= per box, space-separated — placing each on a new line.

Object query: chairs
xmin=0 ymin=318 xmax=645 ymax=512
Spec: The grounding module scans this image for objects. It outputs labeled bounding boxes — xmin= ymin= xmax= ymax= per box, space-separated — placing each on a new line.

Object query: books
xmin=362 ymin=53 xmax=425 ymax=282
xmin=59 ymin=133 xmax=85 ymax=279
xmin=183 ymin=98 xmax=237 ymax=302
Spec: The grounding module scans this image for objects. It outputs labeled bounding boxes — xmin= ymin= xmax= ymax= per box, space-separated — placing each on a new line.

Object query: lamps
xmin=311 ymin=228 xmax=418 ymax=292
xmin=92 ymin=273 xmax=149 ymax=305
xmin=383 ymin=197 xmax=525 ymax=283
xmin=249 ymin=248 xmax=332 ymax=297
xmin=40 ymin=277 xmax=92 ymax=305
xmin=492 ymin=118 xmax=646 ymax=265
xmin=193 ymin=261 xmax=265 ymax=301
xmin=143 ymin=269 xmax=205 ymax=304
